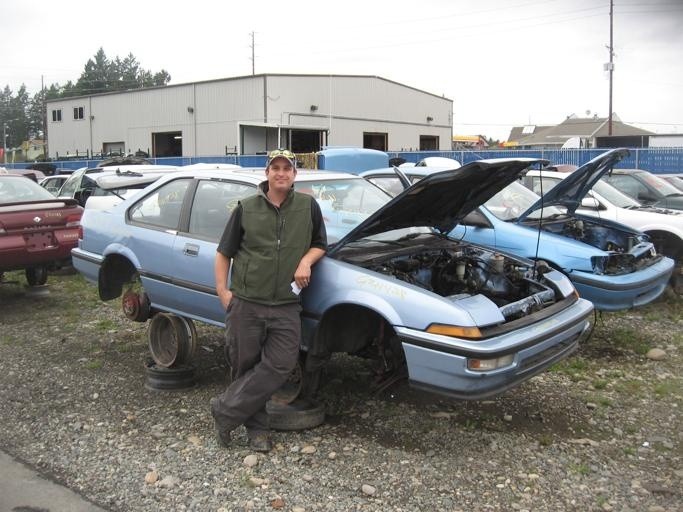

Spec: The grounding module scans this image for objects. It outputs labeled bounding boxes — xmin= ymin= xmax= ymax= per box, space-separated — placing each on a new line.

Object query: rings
xmin=303 ymin=279 xmax=306 ymax=281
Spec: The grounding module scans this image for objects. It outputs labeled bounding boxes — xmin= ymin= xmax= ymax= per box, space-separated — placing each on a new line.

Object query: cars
xmin=317 ymin=148 xmax=683 ymax=310
xmin=0 ymin=163 xmax=244 ymax=285
xmin=71 ymin=158 xmax=597 ymax=400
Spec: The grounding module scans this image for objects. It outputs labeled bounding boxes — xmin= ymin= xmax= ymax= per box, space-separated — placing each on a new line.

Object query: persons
xmin=209 ymin=145 xmax=328 ymax=452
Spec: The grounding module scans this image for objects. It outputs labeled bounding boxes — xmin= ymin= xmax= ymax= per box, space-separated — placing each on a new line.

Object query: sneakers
xmin=210 ymin=396 xmax=233 ymax=448
xmin=249 ymin=431 xmax=272 ymax=453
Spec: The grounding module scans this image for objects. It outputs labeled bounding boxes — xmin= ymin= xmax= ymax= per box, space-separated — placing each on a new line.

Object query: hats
xmin=266 ymin=149 xmax=297 ymax=172
